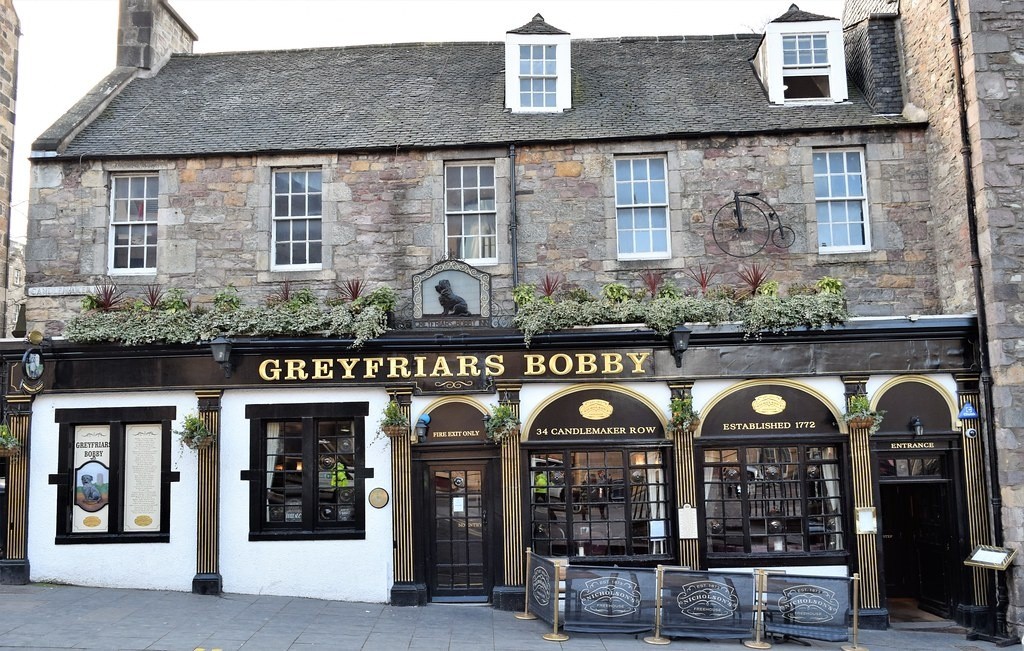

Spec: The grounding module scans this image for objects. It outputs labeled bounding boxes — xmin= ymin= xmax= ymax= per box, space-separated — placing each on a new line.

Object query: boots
xmin=582 ymin=510 xmax=586 ymax=520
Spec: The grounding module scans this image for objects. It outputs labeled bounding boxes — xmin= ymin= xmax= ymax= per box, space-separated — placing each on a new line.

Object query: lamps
xmin=910 ymin=415 xmax=925 ymax=437
xmin=415 ymin=418 xmax=427 ymax=443
xmin=669 ymin=322 xmax=693 ymax=368
xmin=208 ymin=333 xmax=233 ymax=379
xmin=481 ymin=412 xmax=491 ymax=426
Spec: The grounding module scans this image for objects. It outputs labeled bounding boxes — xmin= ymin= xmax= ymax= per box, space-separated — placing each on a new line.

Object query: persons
xmin=534 ymin=472 xmax=613 ymax=521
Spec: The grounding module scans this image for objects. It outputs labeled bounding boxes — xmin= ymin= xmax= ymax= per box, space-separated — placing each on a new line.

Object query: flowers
xmin=484 ymin=403 xmax=522 ymax=446
xmin=666 ymin=395 xmax=701 ymax=432
xmin=0 ymin=423 xmax=23 ymax=451
xmin=833 ymin=390 xmax=888 ymax=437
xmin=367 ymin=399 xmax=408 ymax=453
xmin=169 ymin=407 xmax=216 ymax=469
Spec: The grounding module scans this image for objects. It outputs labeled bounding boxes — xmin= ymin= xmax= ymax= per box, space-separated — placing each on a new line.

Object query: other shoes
xmin=601 ymin=514 xmax=606 ymax=519
